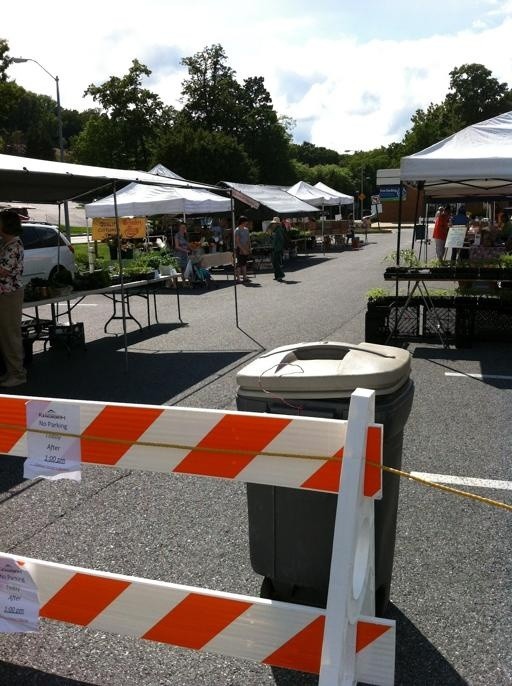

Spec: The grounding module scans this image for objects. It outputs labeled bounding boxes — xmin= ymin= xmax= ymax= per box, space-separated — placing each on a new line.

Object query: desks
xmin=21 ymin=273 xmax=185 ymax=358
xmin=384 ymin=279 xmax=512 ymax=353
xmin=201 ymin=252 xmax=234 ymax=281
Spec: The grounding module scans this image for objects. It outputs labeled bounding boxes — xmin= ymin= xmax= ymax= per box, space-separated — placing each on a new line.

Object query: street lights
xmin=13 ymin=57 xmax=65 ymax=161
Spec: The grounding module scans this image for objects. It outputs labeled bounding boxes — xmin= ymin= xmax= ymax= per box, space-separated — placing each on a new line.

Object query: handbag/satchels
xmin=240 ymin=244 xmax=249 ymax=254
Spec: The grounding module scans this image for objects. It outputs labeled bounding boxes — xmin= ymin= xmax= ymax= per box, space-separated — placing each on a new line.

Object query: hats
xmin=271 ymin=217 xmax=281 ymax=224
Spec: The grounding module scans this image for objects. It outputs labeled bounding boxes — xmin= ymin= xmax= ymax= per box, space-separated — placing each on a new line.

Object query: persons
xmin=269 ymin=216 xmax=286 ymax=281
xmin=433 ymin=207 xmax=453 ymax=263
xmin=345 ymin=213 xmax=354 ymax=245
xmin=494 ymin=210 xmax=512 ymax=234
xmin=234 ymin=216 xmax=253 ymax=283
xmin=1 ymin=209 xmax=29 ymax=388
xmin=432 ymin=203 xmax=470 ymax=225
xmin=174 ymin=222 xmax=192 ymax=284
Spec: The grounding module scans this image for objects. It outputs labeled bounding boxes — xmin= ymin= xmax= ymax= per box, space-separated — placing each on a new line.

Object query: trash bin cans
xmin=236 ymin=341 xmax=415 ymax=614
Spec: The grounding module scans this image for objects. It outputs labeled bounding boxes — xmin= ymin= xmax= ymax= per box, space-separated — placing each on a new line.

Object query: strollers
xmin=186 ymin=250 xmax=211 ymax=290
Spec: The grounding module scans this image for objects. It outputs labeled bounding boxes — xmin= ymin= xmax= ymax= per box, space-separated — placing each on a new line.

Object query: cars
xmin=21 ymin=220 xmax=78 ymax=291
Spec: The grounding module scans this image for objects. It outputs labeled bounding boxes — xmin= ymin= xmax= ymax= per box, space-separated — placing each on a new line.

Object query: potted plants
xmin=366 ymin=288 xmax=512 ymax=312
xmin=382 ymin=249 xmax=512 ymax=280
xmin=30 ymin=253 xmax=177 ymax=298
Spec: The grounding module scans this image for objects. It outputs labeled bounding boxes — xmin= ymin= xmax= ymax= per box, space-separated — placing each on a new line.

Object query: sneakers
xmin=243 ymin=279 xmax=251 ymax=283
